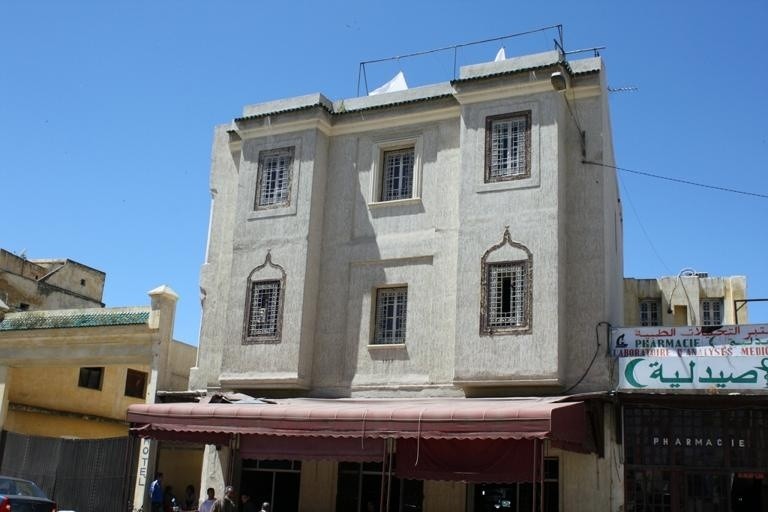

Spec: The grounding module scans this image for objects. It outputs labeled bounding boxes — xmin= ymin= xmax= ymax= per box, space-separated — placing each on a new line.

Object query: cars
xmin=0 ymin=476 xmax=57 ymax=512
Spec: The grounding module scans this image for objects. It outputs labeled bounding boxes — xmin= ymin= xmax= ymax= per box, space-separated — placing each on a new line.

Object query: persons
xmin=259 ymin=501 xmax=270 ymax=511
xmin=210 ymin=484 xmax=236 ymax=511
xmin=163 ymin=485 xmax=175 ymax=511
xmin=239 ymin=492 xmax=258 ymax=511
xmin=199 ymin=488 xmax=218 ymax=511
xmin=183 ymin=485 xmax=196 ymax=511
xmin=149 ymin=473 xmax=163 ymax=511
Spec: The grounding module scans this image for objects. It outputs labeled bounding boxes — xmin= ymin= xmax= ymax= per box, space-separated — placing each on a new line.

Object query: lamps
xmin=550 ymin=72 xmax=585 ymax=156
